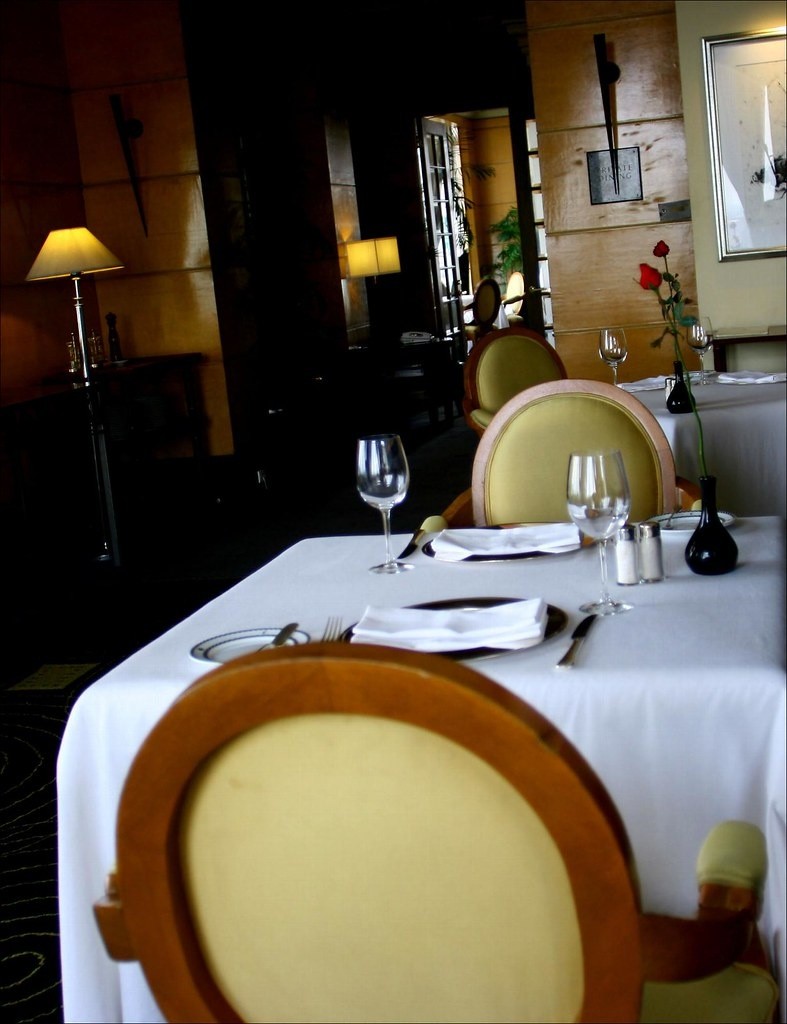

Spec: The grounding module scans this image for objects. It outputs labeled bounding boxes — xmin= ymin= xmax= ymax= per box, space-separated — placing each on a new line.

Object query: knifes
xmin=567 ymin=448 xmax=634 ymax=616
xmin=397 ymin=529 xmax=424 ymax=559
xmin=556 ymin=614 xmax=597 ymax=666
xmin=257 ymin=623 xmax=299 ymax=653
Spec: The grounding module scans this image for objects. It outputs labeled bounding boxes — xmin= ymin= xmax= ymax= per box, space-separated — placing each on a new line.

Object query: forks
xmin=320 ymin=617 xmax=342 ymax=642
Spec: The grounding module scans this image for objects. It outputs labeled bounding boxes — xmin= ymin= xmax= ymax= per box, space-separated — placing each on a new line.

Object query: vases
xmin=666 ymin=361 xmax=695 ymax=413
xmin=685 ymin=475 xmax=738 ymax=576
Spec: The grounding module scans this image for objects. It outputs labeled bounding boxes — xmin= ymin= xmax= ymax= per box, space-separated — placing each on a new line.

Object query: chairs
xmin=418 ymin=379 xmax=701 ymax=528
xmin=95 ymin=641 xmax=779 ymax=1023
xmin=461 ymin=327 xmax=567 ymax=440
xmin=462 ymin=279 xmax=501 ymax=325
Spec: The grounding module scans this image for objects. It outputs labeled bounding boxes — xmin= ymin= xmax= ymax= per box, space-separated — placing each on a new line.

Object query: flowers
xmin=640 ymin=241 xmax=718 ymax=477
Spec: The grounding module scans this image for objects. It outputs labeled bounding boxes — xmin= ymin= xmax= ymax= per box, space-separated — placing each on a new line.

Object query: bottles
xmin=616 ymin=525 xmax=638 ymax=586
xmin=665 ymin=378 xmax=677 ymax=402
xmin=616 ymin=525 xmax=639 ymax=586
xmin=639 ymin=522 xmax=665 ymax=583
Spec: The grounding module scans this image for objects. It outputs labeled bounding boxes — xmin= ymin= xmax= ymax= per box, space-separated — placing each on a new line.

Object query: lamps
xmin=24 ymin=227 xmax=125 ymax=388
xmin=337 ymin=236 xmax=401 ymax=281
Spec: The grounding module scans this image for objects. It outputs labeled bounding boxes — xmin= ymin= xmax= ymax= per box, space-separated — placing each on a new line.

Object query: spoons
xmin=663 ymin=506 xmax=682 ymax=529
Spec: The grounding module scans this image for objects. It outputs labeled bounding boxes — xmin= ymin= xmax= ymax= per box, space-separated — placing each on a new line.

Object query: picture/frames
xmin=701 ymin=25 xmax=787 ymax=262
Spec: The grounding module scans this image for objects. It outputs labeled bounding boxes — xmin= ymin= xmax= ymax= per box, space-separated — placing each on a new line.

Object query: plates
xmin=338 ymin=597 xmax=569 ymax=663
xmin=646 ymin=510 xmax=735 ymax=533
xmin=421 ymin=522 xmax=598 ymax=563
xmin=187 ymin=628 xmax=311 ymax=669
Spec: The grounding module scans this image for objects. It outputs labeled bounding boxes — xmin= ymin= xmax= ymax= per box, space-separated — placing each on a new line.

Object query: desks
xmin=615 ymin=371 xmax=787 ymax=516
xmin=346 ymin=336 xmax=457 ymax=427
xmin=710 ymin=335 xmax=787 ymax=372
xmin=442 ymin=295 xmax=474 ymax=330
xmin=56 ymin=515 xmax=786 ymax=1024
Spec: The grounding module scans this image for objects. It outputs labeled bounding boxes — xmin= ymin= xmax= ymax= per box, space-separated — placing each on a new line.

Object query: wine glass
xmin=687 ymin=317 xmax=715 ymax=385
xmin=566 ymin=451 xmax=633 ymax=614
xmin=599 ymin=328 xmax=628 ymax=387
xmin=356 ymin=433 xmax=415 ymax=574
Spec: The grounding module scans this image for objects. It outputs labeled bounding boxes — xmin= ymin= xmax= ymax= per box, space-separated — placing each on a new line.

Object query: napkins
xmin=616 ymin=374 xmax=676 ymax=393
xmin=430 ymin=523 xmax=581 ymax=562
xmin=350 ymin=597 xmax=548 ymax=654
xmin=716 ymin=372 xmax=786 ymax=385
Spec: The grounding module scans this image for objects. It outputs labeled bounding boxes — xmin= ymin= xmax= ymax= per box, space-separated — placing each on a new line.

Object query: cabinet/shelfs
xmin=0 ymin=353 xmax=208 ymax=683
